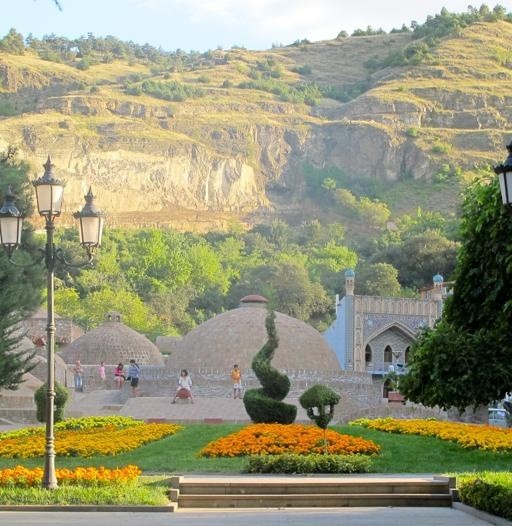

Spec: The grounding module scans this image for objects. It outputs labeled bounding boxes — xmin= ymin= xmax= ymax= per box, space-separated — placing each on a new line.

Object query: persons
xmin=170 ymin=368 xmax=194 ymax=405
xmin=127 ymin=359 xmax=141 ymax=398
xmin=114 ymin=363 xmax=126 ymax=391
xmin=74 ymin=360 xmax=84 ymax=393
xmin=98 ymin=362 xmax=106 ymax=381
xmin=231 ymin=364 xmax=242 ymax=399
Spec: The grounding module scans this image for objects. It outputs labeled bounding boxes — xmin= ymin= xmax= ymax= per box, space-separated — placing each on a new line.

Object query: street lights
xmin=0 ymin=155 xmax=102 ymax=489
